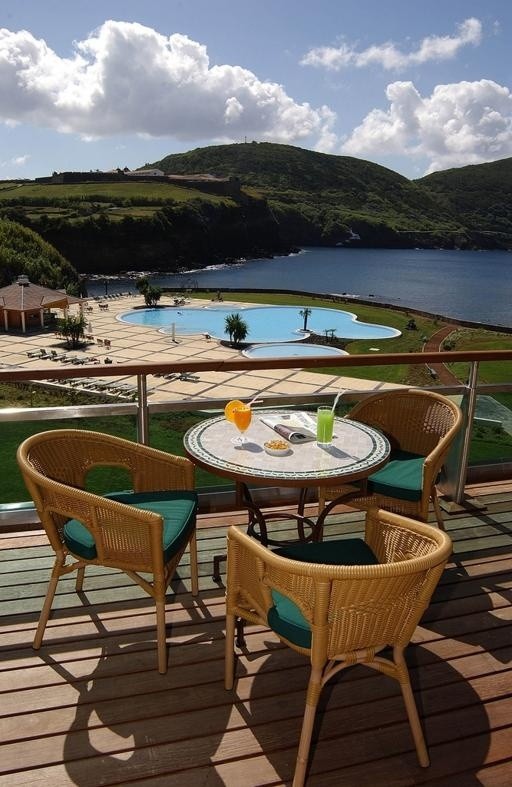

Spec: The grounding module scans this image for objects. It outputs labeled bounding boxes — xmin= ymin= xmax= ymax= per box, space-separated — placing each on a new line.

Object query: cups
xmin=316 ymin=406 xmax=335 ymax=449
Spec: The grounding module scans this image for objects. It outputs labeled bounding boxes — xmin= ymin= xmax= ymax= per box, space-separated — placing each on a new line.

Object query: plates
xmin=263 ymin=440 xmax=292 ymax=456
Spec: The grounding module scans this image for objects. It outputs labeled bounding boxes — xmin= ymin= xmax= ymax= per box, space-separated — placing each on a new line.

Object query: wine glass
xmin=231 ymin=406 xmax=251 ymax=445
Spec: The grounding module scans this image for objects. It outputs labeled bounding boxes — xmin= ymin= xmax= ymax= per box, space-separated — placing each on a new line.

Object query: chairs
xmin=80 ymin=287 xmax=134 ymax=313
xmin=315 ymin=387 xmax=471 ymax=586
xmin=224 ymin=505 xmax=455 ymax=787
xmin=25 ymin=347 xmax=200 ymax=397
xmin=15 ymin=428 xmax=200 ymax=676
